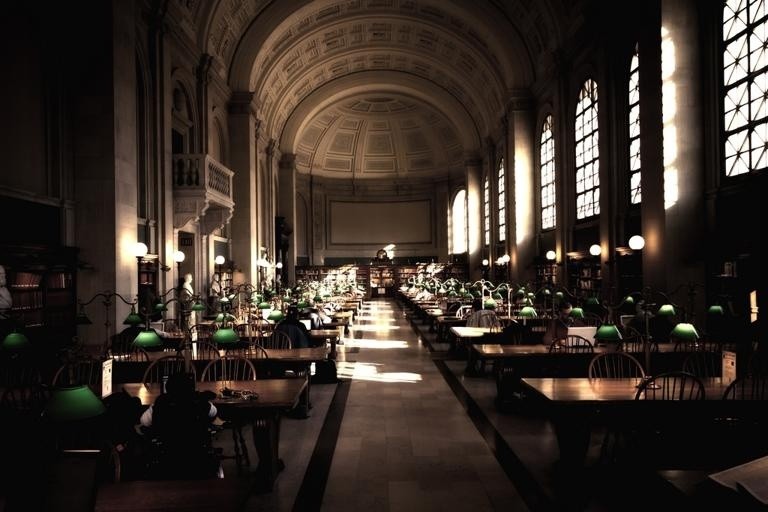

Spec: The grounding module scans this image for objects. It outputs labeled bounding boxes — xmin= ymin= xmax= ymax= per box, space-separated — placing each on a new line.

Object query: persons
xmin=541 ymin=301 xmax=587 ymax=344
xmin=140 ymin=372 xmax=218 ymax=464
xmin=272 ymin=298 xmax=323 ymax=379
xmin=461 ymin=299 xmax=501 ymax=377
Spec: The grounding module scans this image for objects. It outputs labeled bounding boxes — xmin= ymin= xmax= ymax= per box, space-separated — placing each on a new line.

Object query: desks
xmin=0 ymin=268 xmax=365 ymax=511
xmin=399 ymin=278 xmax=767 ymax=511
